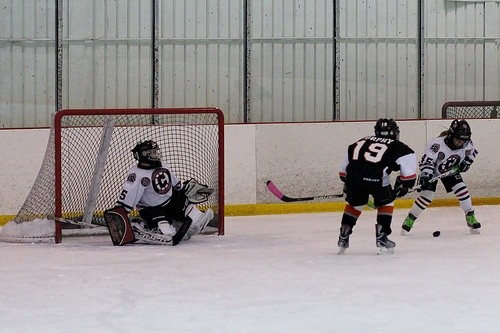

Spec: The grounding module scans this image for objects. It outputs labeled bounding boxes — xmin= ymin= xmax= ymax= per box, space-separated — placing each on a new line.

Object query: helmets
xmin=374 ymin=118 xmax=401 ymax=140
xmin=132 ymin=140 xmax=163 ymax=167
xmin=447 ymin=118 xmax=471 ymax=149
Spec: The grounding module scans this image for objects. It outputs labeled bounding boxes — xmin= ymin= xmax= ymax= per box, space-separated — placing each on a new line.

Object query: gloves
xmin=419 ymin=172 xmax=433 ymax=189
xmin=394 ymin=175 xmax=415 ymax=198
xmin=182 ymin=178 xmax=215 ymax=203
xmin=458 ymin=160 xmax=471 ymax=172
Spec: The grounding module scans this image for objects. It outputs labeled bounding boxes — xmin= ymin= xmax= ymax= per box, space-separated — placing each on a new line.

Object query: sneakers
xmin=401 ymin=212 xmax=418 ymax=236
xmin=337 ymin=227 xmax=353 ymax=254
xmin=374 ymin=224 xmax=396 ymax=255
xmin=465 ymin=210 xmax=481 ymax=234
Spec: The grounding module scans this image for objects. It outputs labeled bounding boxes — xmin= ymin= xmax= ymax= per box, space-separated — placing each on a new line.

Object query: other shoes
xmin=128 ymin=218 xmax=143 ymax=233
xmin=174 ymin=221 xmax=191 ymax=241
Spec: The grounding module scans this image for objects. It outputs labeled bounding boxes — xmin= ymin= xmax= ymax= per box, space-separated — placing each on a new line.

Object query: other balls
xmin=433 ymin=231 xmax=440 ymax=237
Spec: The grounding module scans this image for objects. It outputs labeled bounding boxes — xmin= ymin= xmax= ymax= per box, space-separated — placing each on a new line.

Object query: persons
xmin=401 ymin=118 xmax=481 ymax=232
xmin=338 ymin=117 xmax=417 ymax=249
xmin=114 ymin=140 xmax=214 ymax=241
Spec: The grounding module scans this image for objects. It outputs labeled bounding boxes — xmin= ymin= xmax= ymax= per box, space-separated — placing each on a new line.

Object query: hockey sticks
xmin=367 ymin=167 xmax=459 ymax=209
xmin=47 ymin=214 xmax=193 ymax=246
xmin=266 ymin=179 xmax=343 ymax=202
